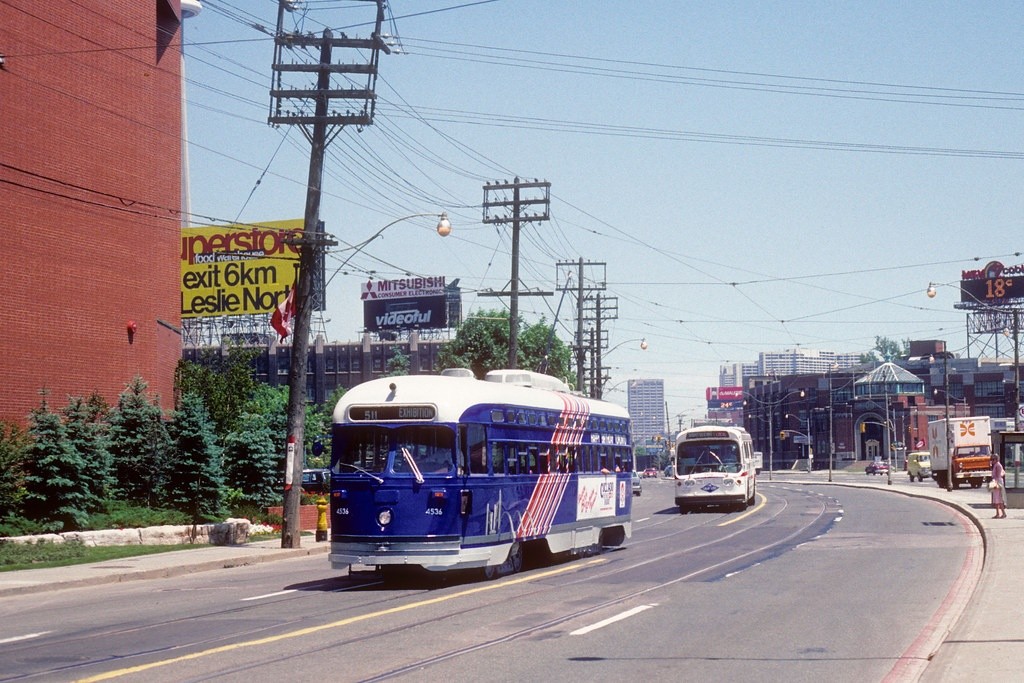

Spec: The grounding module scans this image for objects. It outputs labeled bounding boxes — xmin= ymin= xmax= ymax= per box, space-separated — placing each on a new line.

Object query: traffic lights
xmin=780 ymin=432 xmax=785 ymax=441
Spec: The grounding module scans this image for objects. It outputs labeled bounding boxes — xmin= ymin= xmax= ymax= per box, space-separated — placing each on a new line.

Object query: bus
xmin=312 ymin=368 xmax=672 ymax=583
xmin=674 ymin=426 xmax=757 ymax=513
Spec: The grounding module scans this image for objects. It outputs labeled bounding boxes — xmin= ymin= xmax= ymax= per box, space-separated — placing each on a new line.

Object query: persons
xmin=722 ymin=446 xmax=737 ymax=462
xmin=989 ymin=453 xmax=1007 ymax=518
xmin=600 ymin=465 xmax=610 ymax=473
xmin=615 ymin=462 xmax=620 ymax=472
xmin=445 ymin=448 xmax=464 ymax=470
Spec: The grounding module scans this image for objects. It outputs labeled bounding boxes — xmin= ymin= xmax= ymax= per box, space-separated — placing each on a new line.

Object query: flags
xmin=271 ymin=276 xmax=298 ymax=344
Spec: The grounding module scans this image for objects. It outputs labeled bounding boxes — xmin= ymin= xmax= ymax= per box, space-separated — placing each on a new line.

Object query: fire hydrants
xmin=314 ymin=495 xmax=330 ymax=542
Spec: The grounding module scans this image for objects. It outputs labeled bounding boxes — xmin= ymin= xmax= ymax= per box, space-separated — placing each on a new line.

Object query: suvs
xmin=906 ymin=453 xmax=932 ymax=482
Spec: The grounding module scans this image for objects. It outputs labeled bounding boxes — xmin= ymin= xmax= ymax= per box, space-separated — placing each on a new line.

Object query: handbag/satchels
xmin=988 ymin=480 xmax=1001 ymax=492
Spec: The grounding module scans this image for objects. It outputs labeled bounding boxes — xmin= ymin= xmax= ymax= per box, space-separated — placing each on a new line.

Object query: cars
xmin=632 ymin=471 xmax=643 ymax=495
xmin=643 ymin=468 xmax=659 ymax=478
xmin=664 ymin=465 xmax=676 ymax=478
xmin=864 ymin=462 xmax=894 ymax=475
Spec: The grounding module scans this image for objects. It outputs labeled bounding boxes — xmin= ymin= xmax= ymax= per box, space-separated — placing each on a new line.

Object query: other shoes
xmin=1001 ymin=514 xmax=1006 ymax=518
xmin=992 ymin=515 xmax=1000 ymax=518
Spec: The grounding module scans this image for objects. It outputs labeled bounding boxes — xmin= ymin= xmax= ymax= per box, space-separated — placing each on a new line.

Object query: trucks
xmin=927 ymin=416 xmax=995 ymax=486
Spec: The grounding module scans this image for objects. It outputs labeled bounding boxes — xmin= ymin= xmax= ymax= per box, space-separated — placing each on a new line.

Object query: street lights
xmin=934 ymin=390 xmax=966 ymax=416
xmin=854 ymin=396 xmax=900 ymax=472
xmin=829 ymin=363 xmax=861 ymax=481
xmin=927 ymin=281 xmax=1020 ymax=488
xmin=942 ymin=340 xmax=957 ymax=490
xmin=742 ymin=390 xmax=805 ymax=481
xmin=281 ymin=213 xmax=454 ymax=549
xmin=597 ymin=337 xmax=648 ymax=401
xmin=786 ymin=414 xmax=811 ymax=474
